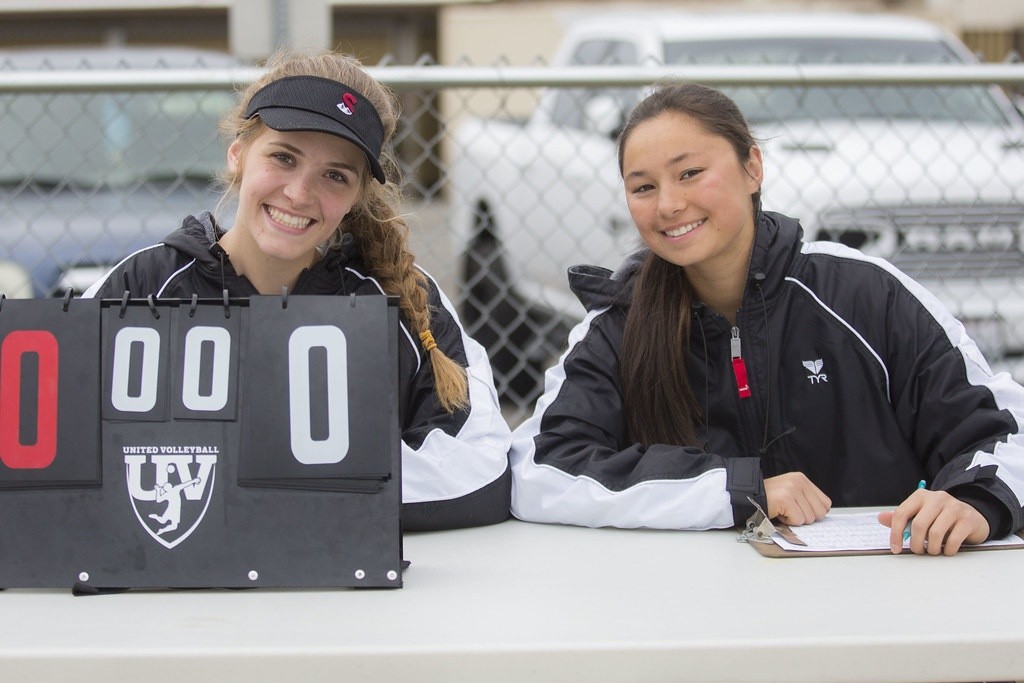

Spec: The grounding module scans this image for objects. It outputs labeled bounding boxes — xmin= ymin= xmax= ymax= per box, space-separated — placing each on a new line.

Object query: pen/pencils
xmin=901 ymin=480 xmax=927 ymax=541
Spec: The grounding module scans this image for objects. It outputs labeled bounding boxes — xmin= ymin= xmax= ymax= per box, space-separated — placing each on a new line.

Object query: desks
xmin=0 ymin=521 xmax=1024 ymax=683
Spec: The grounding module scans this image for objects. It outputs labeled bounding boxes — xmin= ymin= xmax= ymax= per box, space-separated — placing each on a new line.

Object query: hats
xmin=244 ymin=75 xmax=386 ymax=185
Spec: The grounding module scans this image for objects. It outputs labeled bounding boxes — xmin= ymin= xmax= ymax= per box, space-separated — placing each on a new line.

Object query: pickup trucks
xmin=445 ymin=10 xmax=1024 ymax=408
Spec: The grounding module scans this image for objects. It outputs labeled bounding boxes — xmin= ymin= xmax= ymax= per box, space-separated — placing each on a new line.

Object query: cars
xmin=0 ymin=47 xmax=249 ymax=298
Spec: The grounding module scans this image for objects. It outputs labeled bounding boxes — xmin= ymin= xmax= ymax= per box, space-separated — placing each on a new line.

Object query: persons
xmin=510 ymin=84 xmax=1024 ymax=556
xmin=82 ymin=54 xmax=511 ymax=535
